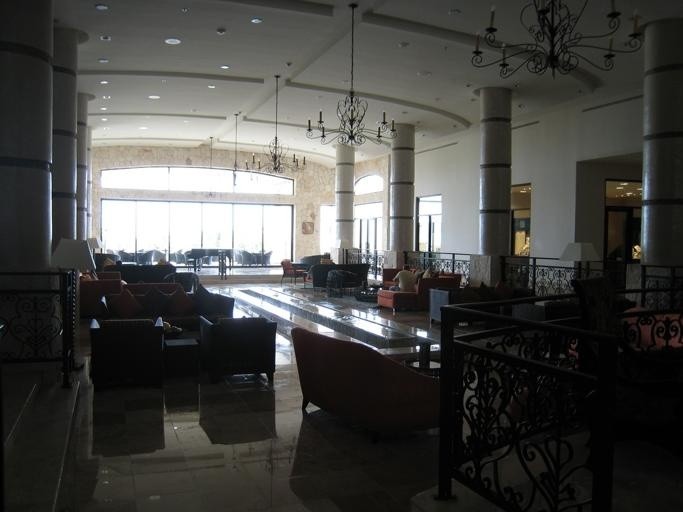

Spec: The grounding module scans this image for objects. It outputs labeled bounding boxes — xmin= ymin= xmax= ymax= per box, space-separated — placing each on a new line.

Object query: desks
xmin=165 ymin=338 xmax=199 ymax=369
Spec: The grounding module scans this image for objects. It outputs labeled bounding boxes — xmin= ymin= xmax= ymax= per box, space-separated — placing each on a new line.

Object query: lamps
xmin=90 ymin=237 xmax=104 ymax=259
xmin=471 ymin=0 xmax=642 ymax=80
xmin=307 ymin=0 xmax=397 ymax=145
xmin=234 ymin=75 xmax=307 ymax=174
xmin=560 ymin=242 xmax=601 ymax=280
xmin=50 ymin=238 xmax=96 ymax=370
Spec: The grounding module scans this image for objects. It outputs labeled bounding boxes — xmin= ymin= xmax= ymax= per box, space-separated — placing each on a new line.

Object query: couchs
xmin=291 ymin=328 xmax=441 ymax=440
xmin=378 ymin=276 xmax=455 ymax=312
xmin=80 ymin=271 xmax=122 ymax=320
xmin=104 ymin=265 xmax=176 ymax=283
xmin=429 ymin=287 xmax=532 ymax=324
xmin=97 ymin=293 xmax=234 ymax=331
xmin=89 ymin=317 xmax=163 ymax=391
xmin=303 ymin=264 xmax=370 ymax=289
xmin=383 ymin=268 xmax=461 ymax=290
xmin=197 ymin=315 xmax=277 ymax=384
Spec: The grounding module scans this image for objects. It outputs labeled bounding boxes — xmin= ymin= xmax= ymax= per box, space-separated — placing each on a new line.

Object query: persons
xmin=390 ymin=265 xmax=416 ymax=291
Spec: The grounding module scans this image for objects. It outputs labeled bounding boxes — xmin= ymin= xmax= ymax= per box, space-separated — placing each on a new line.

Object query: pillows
xmin=110 ymin=284 xmax=219 ymax=318
xmin=414 ymin=267 xmax=439 ymax=285
xmin=459 ymin=280 xmax=510 ymax=302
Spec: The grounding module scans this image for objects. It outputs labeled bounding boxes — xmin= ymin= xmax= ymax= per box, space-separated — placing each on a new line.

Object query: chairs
xmin=281 ymin=261 xmax=306 ymax=285
xmin=107 ymin=249 xmax=272 ymax=280
